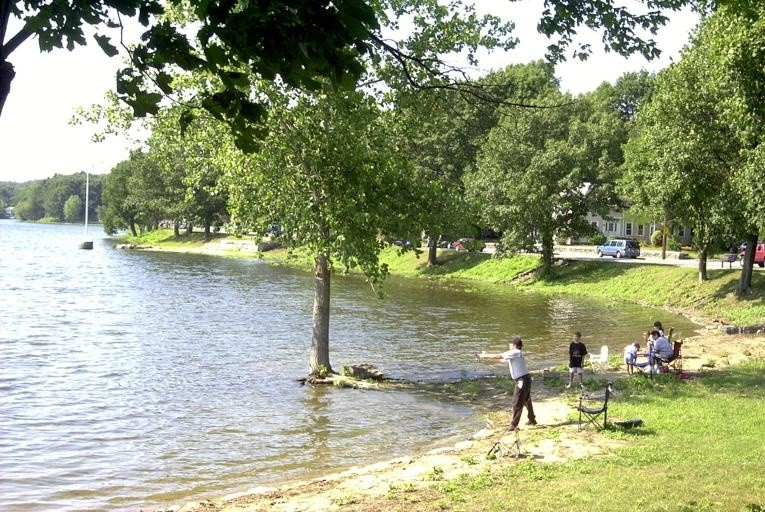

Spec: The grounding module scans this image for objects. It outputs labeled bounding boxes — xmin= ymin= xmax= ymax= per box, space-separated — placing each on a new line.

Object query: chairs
xmin=644 ymin=327 xmax=688 ymax=377
xmin=589 ymin=344 xmax=610 ymax=372
xmin=575 ymin=380 xmax=613 ymax=432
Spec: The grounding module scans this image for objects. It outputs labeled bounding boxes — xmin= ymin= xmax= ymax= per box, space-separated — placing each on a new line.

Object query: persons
xmin=643 ymin=330 xmax=655 ymax=374
xmin=566 ymin=331 xmax=590 ymax=390
xmin=654 ymin=320 xmax=665 ymax=338
xmin=623 ymin=341 xmax=639 ymax=374
xmin=476 ymin=338 xmax=537 ymax=432
xmin=645 ymin=331 xmax=675 ymax=375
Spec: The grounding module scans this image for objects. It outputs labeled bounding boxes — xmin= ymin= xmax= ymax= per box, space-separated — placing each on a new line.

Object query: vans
xmin=596 ymin=240 xmax=640 ymax=258
xmin=426 ymin=234 xmax=448 ymax=248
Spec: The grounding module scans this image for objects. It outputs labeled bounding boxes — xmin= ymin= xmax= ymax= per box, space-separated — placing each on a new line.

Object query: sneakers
xmin=509 ymin=424 xmax=515 ymax=431
xmin=580 ymin=383 xmax=584 ymax=388
xmin=525 ymin=420 xmax=536 ymax=425
xmin=644 ymin=368 xmax=661 ymax=374
xmin=567 ymin=382 xmax=573 ymax=388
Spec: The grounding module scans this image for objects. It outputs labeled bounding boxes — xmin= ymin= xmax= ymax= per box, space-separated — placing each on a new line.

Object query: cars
xmin=395 ymin=238 xmax=421 ymax=248
xmin=740 ymin=241 xmax=765 ymax=268
xmin=450 ymin=238 xmax=483 ymax=252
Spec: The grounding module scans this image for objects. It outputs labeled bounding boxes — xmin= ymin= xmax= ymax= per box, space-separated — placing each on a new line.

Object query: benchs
xmin=721 ymin=254 xmax=737 ymax=269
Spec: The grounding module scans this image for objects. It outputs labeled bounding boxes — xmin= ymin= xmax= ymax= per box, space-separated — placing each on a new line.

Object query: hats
xmin=509 ymin=339 xmax=522 ymax=347
